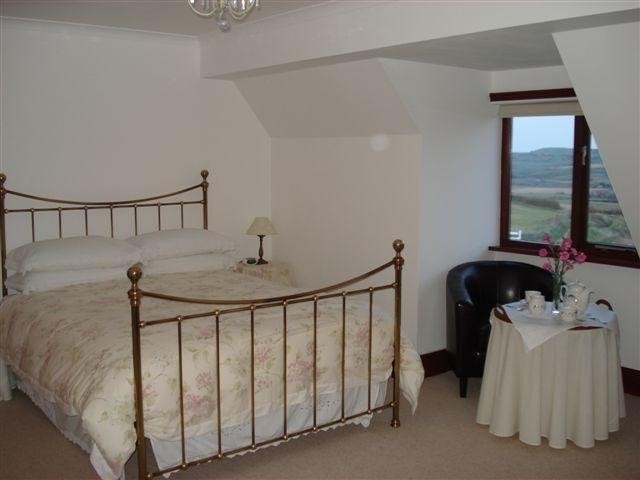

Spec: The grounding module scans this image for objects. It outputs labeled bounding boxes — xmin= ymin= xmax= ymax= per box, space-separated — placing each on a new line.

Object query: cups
xmin=524 ymin=289 xmax=546 ymax=316
xmin=558 ymin=307 xmax=577 ymax=321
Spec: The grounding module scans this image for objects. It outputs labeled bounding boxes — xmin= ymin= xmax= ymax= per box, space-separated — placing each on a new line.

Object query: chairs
xmin=446 ymin=260 xmax=562 ymax=398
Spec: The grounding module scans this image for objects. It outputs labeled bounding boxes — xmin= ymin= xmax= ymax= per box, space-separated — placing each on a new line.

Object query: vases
xmin=552 ymin=275 xmax=562 ymax=313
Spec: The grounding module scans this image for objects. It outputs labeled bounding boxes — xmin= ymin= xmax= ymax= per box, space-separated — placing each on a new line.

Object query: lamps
xmin=245 ymin=214 xmax=276 ymax=265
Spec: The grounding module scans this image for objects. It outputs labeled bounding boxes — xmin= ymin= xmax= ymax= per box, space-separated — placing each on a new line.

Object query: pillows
xmin=5 ymin=231 xmax=240 ymax=293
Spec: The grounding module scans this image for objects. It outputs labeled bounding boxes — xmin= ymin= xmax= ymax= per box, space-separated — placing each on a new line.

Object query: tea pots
xmin=559 ymin=280 xmax=596 ymax=316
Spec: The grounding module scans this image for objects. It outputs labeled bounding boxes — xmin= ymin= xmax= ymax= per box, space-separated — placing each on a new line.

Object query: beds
xmin=0 ymin=165 xmax=404 ymax=480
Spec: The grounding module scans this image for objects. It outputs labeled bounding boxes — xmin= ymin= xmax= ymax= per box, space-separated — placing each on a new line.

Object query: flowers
xmin=537 ymin=232 xmax=586 ymax=308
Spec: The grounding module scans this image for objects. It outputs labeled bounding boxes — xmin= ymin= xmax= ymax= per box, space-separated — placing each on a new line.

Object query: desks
xmin=237 ymin=260 xmax=297 ymax=290
xmin=487 ymin=305 xmax=620 ymax=444
